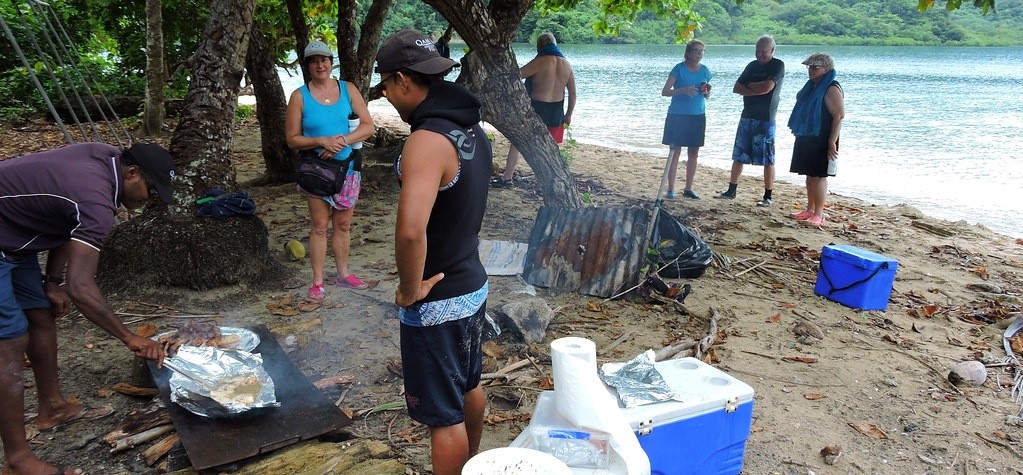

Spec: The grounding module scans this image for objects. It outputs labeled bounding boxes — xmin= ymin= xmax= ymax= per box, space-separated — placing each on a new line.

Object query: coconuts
xmin=285 ymin=240 xmax=305 ymax=262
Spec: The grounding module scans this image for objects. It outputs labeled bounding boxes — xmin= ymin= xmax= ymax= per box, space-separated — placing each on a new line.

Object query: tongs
xmin=163 ymin=359 xmax=215 ymax=391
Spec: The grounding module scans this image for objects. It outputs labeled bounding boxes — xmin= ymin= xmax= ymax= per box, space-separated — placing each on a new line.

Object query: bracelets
xmin=341 ymin=134 xmax=347 ymax=144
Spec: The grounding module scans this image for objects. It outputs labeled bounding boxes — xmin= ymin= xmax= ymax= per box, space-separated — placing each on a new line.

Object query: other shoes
xmin=667 ymin=191 xmax=675 ymax=198
xmin=712 ymin=193 xmax=733 ymax=199
xmin=756 ymin=198 xmax=772 ymax=207
xmin=491 ymin=177 xmax=513 ymax=187
xmin=336 ymin=273 xmax=368 ymax=289
xmin=683 ymin=189 xmax=698 ymax=198
xmin=309 ymin=283 xmax=325 ymax=298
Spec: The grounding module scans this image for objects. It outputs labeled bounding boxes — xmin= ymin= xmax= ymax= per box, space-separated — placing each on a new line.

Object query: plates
xmin=461 ymin=447 xmax=574 ymax=475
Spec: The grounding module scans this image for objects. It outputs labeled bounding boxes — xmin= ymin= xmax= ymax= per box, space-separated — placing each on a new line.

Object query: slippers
xmin=54 ymin=464 xmax=65 ymax=475
xmin=39 ymin=404 xmax=116 ymax=433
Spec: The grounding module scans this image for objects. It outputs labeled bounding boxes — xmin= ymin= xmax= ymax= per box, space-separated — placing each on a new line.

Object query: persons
xmin=0 ymin=142 xmax=177 ymax=475
xmin=661 ymin=39 xmax=713 ymax=199
xmin=789 ymin=53 xmax=845 ymax=225
xmin=491 ymin=34 xmax=576 ymax=187
xmin=714 ymin=36 xmax=786 ymax=207
xmin=285 ymin=40 xmax=375 ymax=302
xmin=375 ymin=27 xmax=494 ymax=475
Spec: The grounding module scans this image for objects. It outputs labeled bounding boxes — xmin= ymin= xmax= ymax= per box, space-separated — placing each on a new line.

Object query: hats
xmin=374 ymin=29 xmax=460 ymax=74
xmin=801 ymin=56 xmax=824 ymax=66
xmin=304 ymin=41 xmax=332 ymax=59
xmin=129 ymin=143 xmax=176 ymax=203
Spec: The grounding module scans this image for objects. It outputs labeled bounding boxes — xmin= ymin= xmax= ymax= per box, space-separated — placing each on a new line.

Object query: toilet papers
xmin=550 ymin=336 xmax=598 ymax=430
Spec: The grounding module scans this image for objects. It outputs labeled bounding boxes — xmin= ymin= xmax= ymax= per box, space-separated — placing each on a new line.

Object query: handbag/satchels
xmin=196 ymin=189 xmax=256 ymax=218
xmin=295 ymin=148 xmax=349 ymax=195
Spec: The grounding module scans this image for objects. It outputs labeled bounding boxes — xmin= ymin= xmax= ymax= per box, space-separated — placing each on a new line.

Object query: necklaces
xmin=324 ymin=98 xmax=331 ymax=104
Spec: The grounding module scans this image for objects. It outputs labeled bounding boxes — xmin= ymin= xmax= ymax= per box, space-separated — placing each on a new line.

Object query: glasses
xmin=139 ymin=169 xmax=160 ymax=203
xmin=806 ymin=65 xmax=824 ymax=69
xmin=375 ymin=75 xmax=395 ymax=97
xmin=691 ymin=48 xmax=706 ymax=54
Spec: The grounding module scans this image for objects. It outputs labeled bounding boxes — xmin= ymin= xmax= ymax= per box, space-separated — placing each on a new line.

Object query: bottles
xmin=348 ymin=112 xmax=362 ymax=150
xmin=948 ymin=361 xmax=987 ymax=384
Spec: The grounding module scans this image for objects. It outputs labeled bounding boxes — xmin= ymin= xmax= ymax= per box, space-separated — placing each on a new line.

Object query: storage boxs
xmin=815 ymin=244 xmax=898 ymax=310
xmin=569 ymin=356 xmax=754 ymax=475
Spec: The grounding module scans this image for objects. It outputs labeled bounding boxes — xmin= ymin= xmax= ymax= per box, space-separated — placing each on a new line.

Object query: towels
xmin=786 ymin=68 xmax=837 ymax=137
xmin=524 ymin=43 xmax=566 ymax=96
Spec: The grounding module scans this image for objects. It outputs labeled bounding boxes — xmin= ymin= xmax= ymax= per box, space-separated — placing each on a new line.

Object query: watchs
xmin=43 ymin=274 xmax=64 ymax=284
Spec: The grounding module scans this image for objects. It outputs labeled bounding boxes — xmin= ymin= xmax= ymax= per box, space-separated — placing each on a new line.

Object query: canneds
xmin=701 ymin=81 xmax=708 ymax=94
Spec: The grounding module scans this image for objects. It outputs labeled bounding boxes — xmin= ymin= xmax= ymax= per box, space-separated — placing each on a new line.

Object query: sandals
xmin=808 ymin=215 xmax=825 ymax=226
xmin=790 ymin=209 xmax=811 ymax=219
